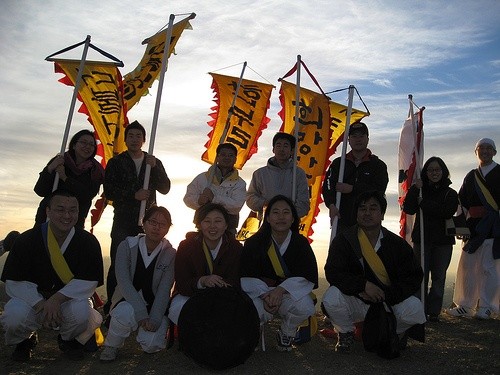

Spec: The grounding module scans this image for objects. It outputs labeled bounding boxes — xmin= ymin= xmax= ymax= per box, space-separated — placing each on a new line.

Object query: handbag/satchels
xmin=362 ymin=300 xmax=400 ymax=359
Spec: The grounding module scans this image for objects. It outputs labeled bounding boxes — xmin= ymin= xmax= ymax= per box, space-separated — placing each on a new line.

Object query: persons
xmin=401 ymin=156 xmax=459 ymax=322
xmin=100 ymin=206 xmax=177 ymax=362
xmin=240 ymin=194 xmax=319 ymax=351
xmin=167 ymin=203 xmax=241 ymax=324
xmin=322 ymin=191 xmax=428 ymax=352
xmin=322 ymin=122 xmax=389 ymax=336
xmin=103 ymin=121 xmax=170 ymax=329
xmin=444 ymin=138 xmax=500 ymax=319
xmin=184 ymin=143 xmax=246 ymax=215
xmin=246 ymin=132 xmax=311 ymax=218
xmin=34 ymin=130 xmax=106 ymax=227
xmin=0 ymin=189 xmax=104 ymax=362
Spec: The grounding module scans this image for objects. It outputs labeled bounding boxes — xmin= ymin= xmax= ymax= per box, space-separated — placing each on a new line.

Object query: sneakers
xmin=475 ymin=308 xmax=493 ymax=319
xmin=276 ymin=330 xmax=292 ymax=347
xmin=445 ymin=306 xmax=473 ymax=317
xmin=334 ymin=331 xmax=353 ymax=352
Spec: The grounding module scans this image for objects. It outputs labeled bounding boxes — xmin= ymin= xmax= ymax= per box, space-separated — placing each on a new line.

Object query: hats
xmin=475 ymin=139 xmax=496 ymax=153
xmin=348 ymin=122 xmax=368 ymax=136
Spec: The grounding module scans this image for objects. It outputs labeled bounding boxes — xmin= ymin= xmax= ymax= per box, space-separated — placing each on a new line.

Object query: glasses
xmin=77 ymin=139 xmax=97 ymax=149
xmin=147 ymin=218 xmax=170 ymax=229
xmin=427 ymin=167 xmax=442 ymax=173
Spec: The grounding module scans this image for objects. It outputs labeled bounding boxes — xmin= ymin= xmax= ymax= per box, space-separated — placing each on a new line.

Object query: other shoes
xmin=14 ymin=333 xmax=37 ymax=361
xmin=100 ymin=346 xmax=117 ymax=361
xmin=58 ymin=333 xmax=82 ymax=360
xmin=427 ymin=314 xmax=438 ymax=321
